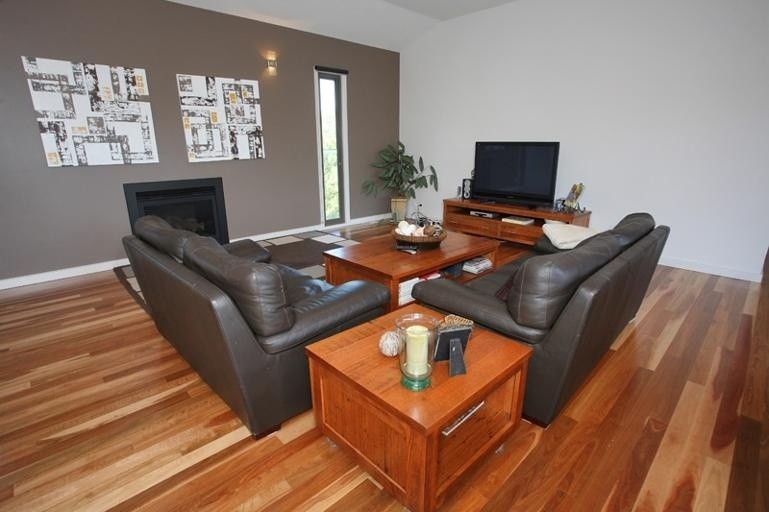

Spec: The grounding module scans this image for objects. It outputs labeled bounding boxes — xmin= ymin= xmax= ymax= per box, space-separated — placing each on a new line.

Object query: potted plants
xmin=360 ymin=139 xmax=438 ymax=226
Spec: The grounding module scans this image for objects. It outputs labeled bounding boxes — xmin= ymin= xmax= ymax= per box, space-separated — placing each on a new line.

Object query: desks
xmin=442 ymin=197 xmax=592 ymax=246
xmin=302 ymin=301 xmax=534 ymax=512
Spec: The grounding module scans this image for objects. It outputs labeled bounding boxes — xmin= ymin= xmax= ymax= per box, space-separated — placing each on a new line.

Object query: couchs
xmin=410 ymin=211 xmax=671 ymax=429
xmin=120 ymin=214 xmax=391 ymax=437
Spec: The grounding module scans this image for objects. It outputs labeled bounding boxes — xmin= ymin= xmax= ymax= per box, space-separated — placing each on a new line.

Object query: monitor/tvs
xmin=473 ymin=142 xmax=559 ymax=209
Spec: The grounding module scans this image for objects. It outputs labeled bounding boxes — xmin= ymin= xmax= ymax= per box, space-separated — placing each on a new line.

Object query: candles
xmin=405 ymin=324 xmax=430 ymax=376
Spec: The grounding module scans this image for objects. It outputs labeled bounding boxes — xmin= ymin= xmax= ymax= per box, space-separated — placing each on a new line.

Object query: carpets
xmin=111 ymin=229 xmax=363 ymax=319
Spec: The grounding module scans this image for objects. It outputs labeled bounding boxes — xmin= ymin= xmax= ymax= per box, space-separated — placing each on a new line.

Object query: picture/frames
xmin=433 ymin=326 xmax=473 ymax=378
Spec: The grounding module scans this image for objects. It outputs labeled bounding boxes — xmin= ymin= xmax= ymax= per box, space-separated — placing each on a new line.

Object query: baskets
xmin=391 ymin=225 xmax=447 ymax=243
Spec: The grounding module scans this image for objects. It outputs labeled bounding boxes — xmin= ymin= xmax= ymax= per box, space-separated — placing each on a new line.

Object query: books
xmin=461 ymin=256 xmax=495 ymax=274
xmin=502 ymin=216 xmax=535 ymax=226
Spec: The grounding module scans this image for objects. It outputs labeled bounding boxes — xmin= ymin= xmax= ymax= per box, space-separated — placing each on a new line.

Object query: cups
xmin=396 ymin=313 xmax=440 ymax=392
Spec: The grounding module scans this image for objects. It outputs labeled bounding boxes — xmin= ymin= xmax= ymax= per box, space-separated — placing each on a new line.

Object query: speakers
xmin=462 ymin=179 xmax=475 ymax=199
